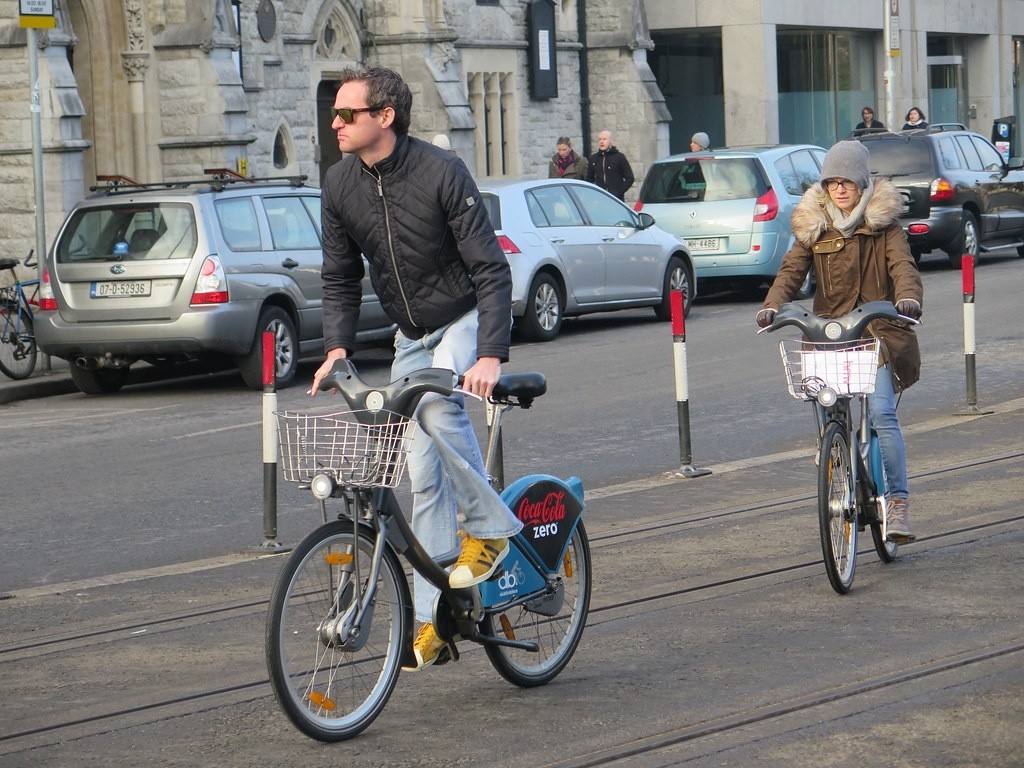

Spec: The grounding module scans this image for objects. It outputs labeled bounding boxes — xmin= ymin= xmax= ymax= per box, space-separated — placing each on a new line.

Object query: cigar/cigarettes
xmin=306 ymin=389 xmax=312 ymax=394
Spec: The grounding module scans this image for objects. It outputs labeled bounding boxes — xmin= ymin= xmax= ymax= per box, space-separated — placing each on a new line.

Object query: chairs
xmin=130 ymin=229 xmax=161 ymax=253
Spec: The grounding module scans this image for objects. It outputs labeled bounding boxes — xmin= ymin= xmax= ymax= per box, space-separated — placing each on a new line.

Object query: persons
xmin=586 ymin=130 xmax=634 ymax=203
xmin=854 ymin=107 xmax=884 ymax=137
xmin=902 ymin=107 xmax=927 ymax=131
xmin=548 ymin=137 xmax=588 ymax=180
xmin=311 ymin=70 xmax=526 ymax=672
xmin=755 ymin=141 xmax=923 ymax=545
xmin=679 ymin=131 xmax=711 ymax=192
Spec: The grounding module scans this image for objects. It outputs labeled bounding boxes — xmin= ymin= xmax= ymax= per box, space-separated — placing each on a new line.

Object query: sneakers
xmin=448 ymin=529 xmax=510 ymax=589
xmin=814 ymin=446 xmax=839 ymax=465
xmin=399 ymin=623 xmax=448 ymax=672
xmin=884 ymin=497 xmax=912 ymax=537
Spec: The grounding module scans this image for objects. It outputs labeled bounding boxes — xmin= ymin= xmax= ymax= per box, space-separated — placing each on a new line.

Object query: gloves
xmin=756 ymin=308 xmax=776 ymax=334
xmin=898 ymin=299 xmax=923 ymax=325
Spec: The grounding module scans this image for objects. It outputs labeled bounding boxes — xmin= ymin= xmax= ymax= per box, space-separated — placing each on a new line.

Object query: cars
xmin=634 ymin=144 xmax=829 ymax=299
xmin=472 ymin=175 xmax=697 ymax=342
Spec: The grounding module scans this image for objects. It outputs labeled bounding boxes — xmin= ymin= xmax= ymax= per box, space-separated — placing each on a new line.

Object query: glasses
xmin=824 ymin=179 xmax=857 ymax=190
xmin=330 ymin=106 xmax=382 ymax=124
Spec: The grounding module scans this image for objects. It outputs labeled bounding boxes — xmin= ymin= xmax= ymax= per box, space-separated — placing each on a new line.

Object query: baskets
xmin=271 ymin=408 xmax=420 ymax=488
xmin=778 ymin=336 xmax=881 ymax=399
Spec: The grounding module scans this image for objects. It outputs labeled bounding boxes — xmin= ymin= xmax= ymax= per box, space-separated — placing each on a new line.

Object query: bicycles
xmin=756 ymin=302 xmax=922 ymax=594
xmin=264 ymin=357 xmax=593 ymax=743
xmin=0 ymin=234 xmax=86 ymax=380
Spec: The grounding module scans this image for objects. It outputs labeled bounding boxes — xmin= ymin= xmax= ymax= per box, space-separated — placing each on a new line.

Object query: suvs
xmin=849 ymin=122 xmax=1024 ymax=268
xmin=32 ymin=176 xmax=395 ymax=397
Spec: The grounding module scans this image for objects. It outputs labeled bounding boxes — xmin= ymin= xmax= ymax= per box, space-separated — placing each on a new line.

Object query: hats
xmin=692 ymin=132 xmax=710 ymax=148
xmin=820 ymin=140 xmax=871 ymax=190
